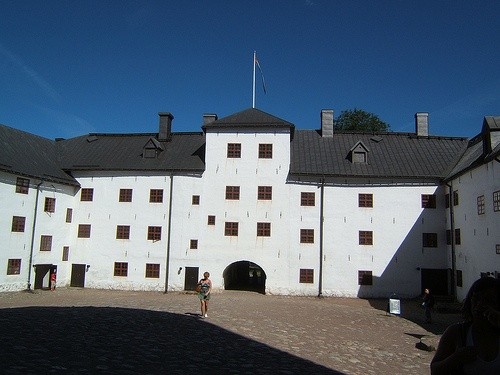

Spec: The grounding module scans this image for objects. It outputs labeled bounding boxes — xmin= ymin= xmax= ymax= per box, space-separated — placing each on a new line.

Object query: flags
xmin=256 ymin=59 xmax=266 ymax=94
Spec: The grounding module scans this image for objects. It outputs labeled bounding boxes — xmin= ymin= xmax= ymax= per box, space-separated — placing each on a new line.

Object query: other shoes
xmin=205 ymin=314 xmax=207 ymax=317
xmin=201 ymin=316 xmax=204 ymax=318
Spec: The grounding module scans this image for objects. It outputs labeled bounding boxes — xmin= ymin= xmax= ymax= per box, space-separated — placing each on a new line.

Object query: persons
xmin=430 ymin=277 xmax=500 ymax=375
xmin=419 ymin=288 xmax=434 ymax=321
xmin=197 ymin=272 xmax=212 ymax=318
xmin=51 ymin=272 xmax=56 ymax=291
